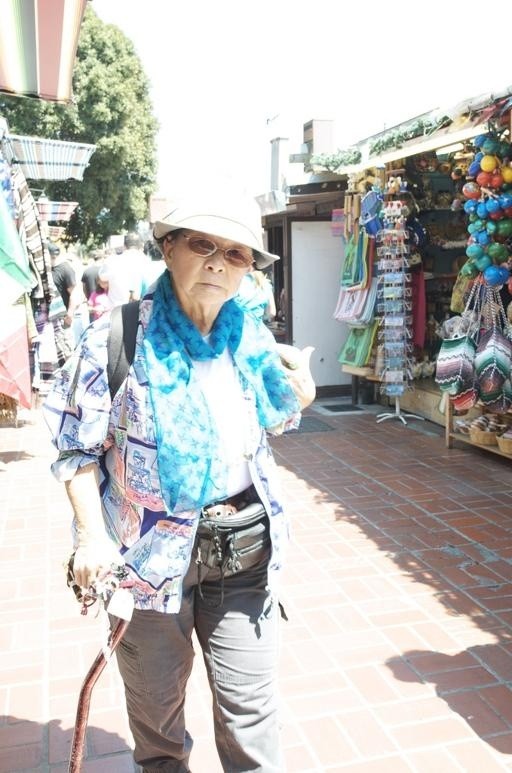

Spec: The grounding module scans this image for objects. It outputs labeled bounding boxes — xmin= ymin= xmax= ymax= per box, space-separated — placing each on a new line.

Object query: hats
xmin=153 ymin=206 xmax=281 ymax=270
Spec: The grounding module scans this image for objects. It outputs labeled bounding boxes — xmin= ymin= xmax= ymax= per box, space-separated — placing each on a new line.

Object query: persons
xmin=237 ymin=266 xmax=276 ymax=322
xmin=50 ymin=232 xmax=165 ymax=369
xmin=48 ymin=189 xmax=317 ymax=773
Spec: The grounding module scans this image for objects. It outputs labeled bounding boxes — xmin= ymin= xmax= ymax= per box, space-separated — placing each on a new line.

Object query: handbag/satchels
xmin=435 ymin=301 xmax=512 ymax=416
xmin=384 ymin=273 xmax=405 ymax=397
xmin=450 ymin=275 xmax=474 ymax=313
xmin=406 ymin=252 xmax=423 ymax=272
xmin=333 ymin=191 xmax=384 ymax=381
xmin=196 ymin=502 xmax=271 ymax=581
xmin=408 ymin=174 xmax=427 ymax=216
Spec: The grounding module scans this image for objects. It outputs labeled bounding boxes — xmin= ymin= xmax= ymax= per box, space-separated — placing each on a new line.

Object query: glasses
xmin=179 ymin=230 xmax=256 ymax=269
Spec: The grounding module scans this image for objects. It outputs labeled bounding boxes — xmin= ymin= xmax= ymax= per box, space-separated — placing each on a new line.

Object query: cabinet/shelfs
xmin=445 ymin=391 xmax=511 ymax=460
xmin=374 ymin=214 xmax=427 ymax=425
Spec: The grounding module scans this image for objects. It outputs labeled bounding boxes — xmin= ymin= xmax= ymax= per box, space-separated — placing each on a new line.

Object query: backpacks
xmin=406 ymin=215 xmax=430 ymax=247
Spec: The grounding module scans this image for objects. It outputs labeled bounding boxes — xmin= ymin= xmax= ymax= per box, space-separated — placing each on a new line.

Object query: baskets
xmin=468 ymin=427 xmax=512 ymax=455
